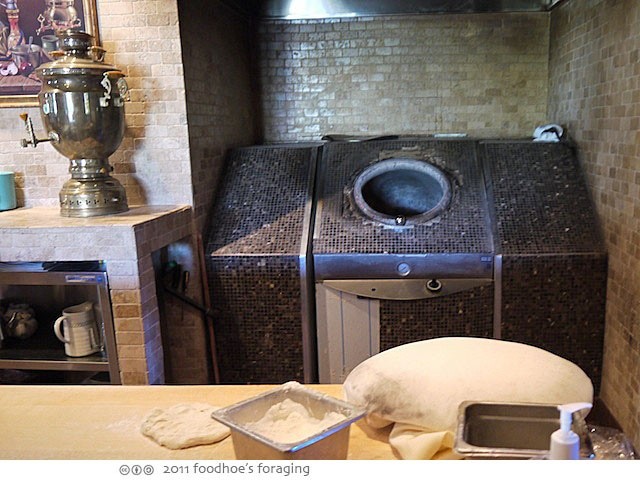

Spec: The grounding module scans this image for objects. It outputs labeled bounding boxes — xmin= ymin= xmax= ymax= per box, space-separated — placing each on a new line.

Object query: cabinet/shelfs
xmin=1 ymin=273 xmax=120 ymax=385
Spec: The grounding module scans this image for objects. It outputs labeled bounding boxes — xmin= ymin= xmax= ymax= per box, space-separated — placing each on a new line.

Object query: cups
xmin=0 ymin=171 xmax=17 ymax=211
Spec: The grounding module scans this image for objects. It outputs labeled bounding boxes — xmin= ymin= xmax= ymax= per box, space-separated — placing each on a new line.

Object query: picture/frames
xmin=0 ymin=0 xmax=102 ymax=107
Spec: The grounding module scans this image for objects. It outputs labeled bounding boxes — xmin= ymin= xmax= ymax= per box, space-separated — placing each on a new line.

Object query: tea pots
xmin=53 ymin=300 xmax=102 ymax=358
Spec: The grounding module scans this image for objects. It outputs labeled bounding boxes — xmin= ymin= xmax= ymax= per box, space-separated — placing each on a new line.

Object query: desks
xmin=0 ymin=384 xmax=639 ymax=461
